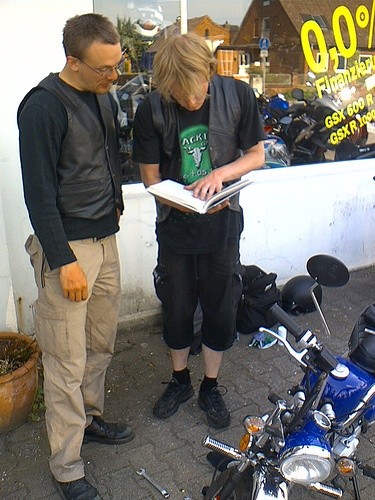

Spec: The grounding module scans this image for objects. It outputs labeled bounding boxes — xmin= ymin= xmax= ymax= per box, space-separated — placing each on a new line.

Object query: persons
xmin=16 ymin=13 xmax=134 ymax=500
xmin=133 ymin=34 xmax=269 ymax=428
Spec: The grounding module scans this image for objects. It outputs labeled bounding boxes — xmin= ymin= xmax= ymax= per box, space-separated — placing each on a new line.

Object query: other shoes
xmin=84 ymin=416 xmax=134 ymax=444
xmin=53 ymin=476 xmax=102 ymax=500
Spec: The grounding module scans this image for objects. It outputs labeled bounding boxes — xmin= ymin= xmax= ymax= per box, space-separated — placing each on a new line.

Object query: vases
xmin=0 ymin=332 xmax=40 ymax=434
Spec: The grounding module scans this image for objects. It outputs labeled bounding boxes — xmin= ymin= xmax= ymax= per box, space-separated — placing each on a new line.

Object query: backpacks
xmin=236 ymin=264 xmax=280 ymax=334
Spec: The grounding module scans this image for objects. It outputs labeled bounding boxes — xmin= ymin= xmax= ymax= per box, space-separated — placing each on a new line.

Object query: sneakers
xmin=153 ymin=378 xmax=194 ymax=418
xmin=197 ymin=384 xmax=231 ymax=428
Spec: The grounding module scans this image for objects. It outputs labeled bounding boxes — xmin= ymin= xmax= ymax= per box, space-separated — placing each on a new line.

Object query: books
xmin=146 ymin=178 xmax=255 ymax=214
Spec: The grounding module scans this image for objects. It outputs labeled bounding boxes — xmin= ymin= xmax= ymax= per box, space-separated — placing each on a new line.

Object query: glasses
xmin=68 ymin=54 xmax=126 ymax=78
